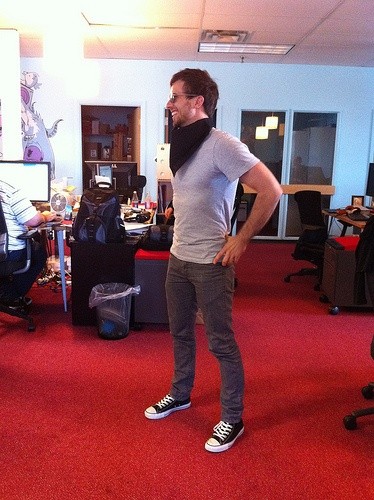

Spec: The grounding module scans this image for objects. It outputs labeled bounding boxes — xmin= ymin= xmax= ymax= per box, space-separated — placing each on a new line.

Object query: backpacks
xmin=71 ymin=182 xmax=127 ymax=244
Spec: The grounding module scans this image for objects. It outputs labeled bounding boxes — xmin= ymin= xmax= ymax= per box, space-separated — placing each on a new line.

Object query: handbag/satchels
xmin=140 ymin=224 xmax=174 ymax=251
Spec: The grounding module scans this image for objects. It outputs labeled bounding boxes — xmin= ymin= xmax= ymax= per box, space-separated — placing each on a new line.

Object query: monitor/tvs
xmin=366 ymin=163 xmax=374 ymax=197
xmin=95 ymin=164 xmax=112 ymax=188
xmin=0 ymin=160 xmax=50 ymax=203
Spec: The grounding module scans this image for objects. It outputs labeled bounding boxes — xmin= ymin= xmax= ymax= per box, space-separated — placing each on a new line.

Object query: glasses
xmin=169 ymin=94 xmax=198 ymax=103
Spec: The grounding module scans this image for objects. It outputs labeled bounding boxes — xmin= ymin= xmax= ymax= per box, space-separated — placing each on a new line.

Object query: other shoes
xmin=8 ymin=297 xmax=32 ymax=309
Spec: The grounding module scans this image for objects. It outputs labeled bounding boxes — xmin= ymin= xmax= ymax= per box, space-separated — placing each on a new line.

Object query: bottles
xmin=132 ymin=191 xmax=138 ymax=208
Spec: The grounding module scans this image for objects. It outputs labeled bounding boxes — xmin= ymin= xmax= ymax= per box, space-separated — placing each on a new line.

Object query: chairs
xmin=284 ymin=190 xmax=329 ymax=292
xmin=0 ymin=201 xmax=48 ymax=332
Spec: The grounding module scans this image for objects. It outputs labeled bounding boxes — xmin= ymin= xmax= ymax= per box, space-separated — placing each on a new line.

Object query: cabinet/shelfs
xmin=79 ymin=103 xmax=141 ymax=205
xmin=319 ymin=235 xmax=374 ymax=315
xmin=133 ymin=248 xmax=171 ymax=331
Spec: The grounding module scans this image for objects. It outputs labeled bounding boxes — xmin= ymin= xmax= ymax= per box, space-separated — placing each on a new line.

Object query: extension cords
xmin=37 ymin=274 xmax=56 ymax=285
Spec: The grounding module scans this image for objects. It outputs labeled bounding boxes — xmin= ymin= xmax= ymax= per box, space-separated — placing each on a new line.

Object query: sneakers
xmin=205 ymin=417 xmax=244 ymax=452
xmin=144 ymin=393 xmax=190 ymax=419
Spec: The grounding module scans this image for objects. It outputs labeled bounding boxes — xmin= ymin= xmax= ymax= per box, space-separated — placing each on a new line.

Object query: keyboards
xmin=348 ymin=212 xmax=368 ymax=222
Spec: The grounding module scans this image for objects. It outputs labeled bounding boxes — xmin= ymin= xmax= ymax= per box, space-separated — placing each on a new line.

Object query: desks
xmin=321 ymin=208 xmax=367 ymax=237
xmin=240 ymin=183 xmax=337 ymax=197
xmin=70 ymin=206 xmax=156 ymax=326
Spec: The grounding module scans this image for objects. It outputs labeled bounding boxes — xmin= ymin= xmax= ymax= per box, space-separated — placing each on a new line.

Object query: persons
xmin=144 ymin=68 xmax=284 ymax=453
xmin=0 ymin=178 xmax=57 ymax=311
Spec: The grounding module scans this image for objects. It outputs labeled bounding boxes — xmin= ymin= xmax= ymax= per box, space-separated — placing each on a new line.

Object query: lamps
xmin=265 ymin=113 xmax=279 ymax=129
xmin=255 ymin=113 xmax=269 ymax=139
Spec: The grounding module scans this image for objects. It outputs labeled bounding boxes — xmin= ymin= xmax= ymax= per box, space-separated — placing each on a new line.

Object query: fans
xmin=51 ymin=190 xmax=69 ymax=219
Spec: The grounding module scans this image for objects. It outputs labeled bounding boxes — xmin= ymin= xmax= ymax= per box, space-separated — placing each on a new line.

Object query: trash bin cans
xmin=92 ymin=282 xmax=132 ymax=340
